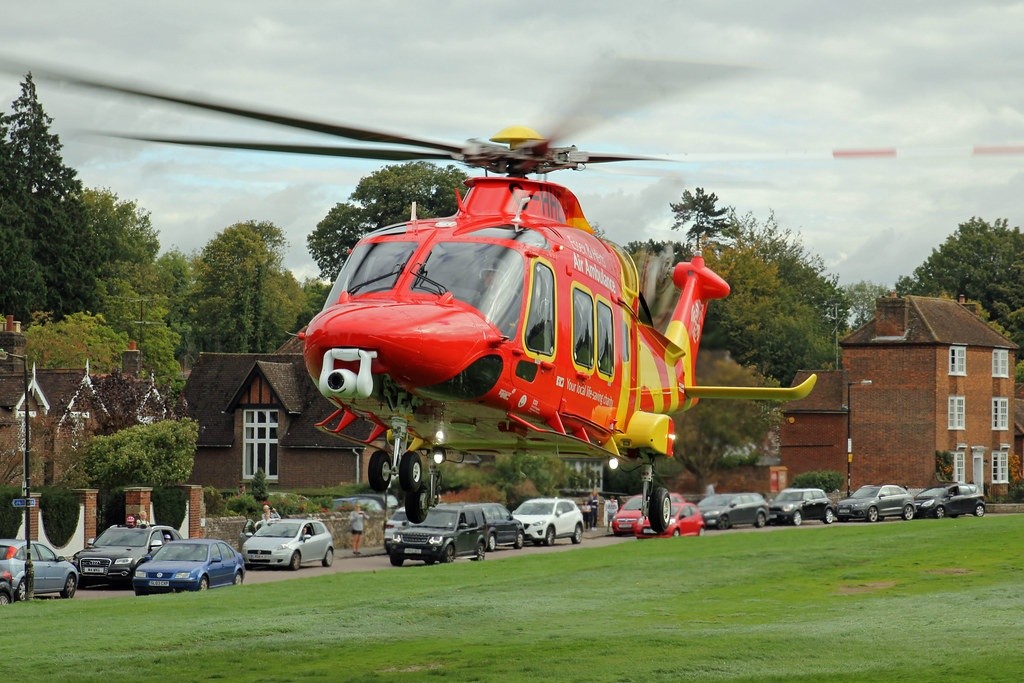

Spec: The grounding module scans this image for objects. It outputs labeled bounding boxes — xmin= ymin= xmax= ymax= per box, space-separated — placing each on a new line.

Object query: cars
xmin=913 ymin=482 xmax=986 ymax=520
xmin=511 ymin=498 xmax=584 ymax=547
xmin=635 ymin=503 xmax=704 ymax=538
xmin=696 ymin=493 xmax=770 ymax=531
xmin=768 ymin=487 xmax=834 ymax=526
xmin=1 ymin=539 xmax=80 ymax=603
xmin=239 ymin=518 xmax=335 ymax=572
xmin=384 ymin=502 xmax=524 ymax=552
xmin=131 ymin=539 xmax=246 ymax=595
xmin=611 ymin=493 xmax=685 ymax=536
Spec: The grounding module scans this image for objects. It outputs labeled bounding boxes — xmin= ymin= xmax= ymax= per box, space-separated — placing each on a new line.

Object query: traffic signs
xmin=12 ymin=499 xmax=36 ymax=507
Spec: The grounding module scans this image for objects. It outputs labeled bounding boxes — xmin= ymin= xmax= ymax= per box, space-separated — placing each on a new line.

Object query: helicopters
xmin=0 ymin=56 xmax=1024 ymax=523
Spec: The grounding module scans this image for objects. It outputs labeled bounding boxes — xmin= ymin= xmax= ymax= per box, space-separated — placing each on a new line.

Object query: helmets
xmin=126 ymin=516 xmax=135 ymax=522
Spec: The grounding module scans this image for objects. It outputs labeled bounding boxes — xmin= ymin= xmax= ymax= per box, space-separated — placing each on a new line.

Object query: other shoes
xmin=353 ymin=551 xmax=361 ymax=554
xmin=591 ymin=527 xmax=597 ymax=531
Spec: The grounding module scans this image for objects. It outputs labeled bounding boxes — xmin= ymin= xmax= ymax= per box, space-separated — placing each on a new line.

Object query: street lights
xmin=0 ymin=348 xmax=35 ymax=601
xmin=847 ymin=380 xmax=873 ymax=496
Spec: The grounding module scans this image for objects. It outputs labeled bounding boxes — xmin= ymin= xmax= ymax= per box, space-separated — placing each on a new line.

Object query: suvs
xmin=389 ymin=505 xmax=489 ymax=566
xmin=836 ymin=485 xmax=916 ymax=523
xmin=73 ymin=522 xmax=183 ymax=590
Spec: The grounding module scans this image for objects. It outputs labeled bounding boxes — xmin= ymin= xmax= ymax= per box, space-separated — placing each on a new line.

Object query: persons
xmin=263 ymin=505 xmax=281 ymax=520
xmin=136 ymin=510 xmax=150 ymax=527
xmin=164 ymin=534 xmax=171 ymax=543
xmin=303 ymin=526 xmax=307 ymax=534
xmin=581 ymin=490 xmax=625 ymax=533
xmin=706 ymin=481 xmax=718 ymax=497
xmin=350 ymin=503 xmax=370 ymax=554
